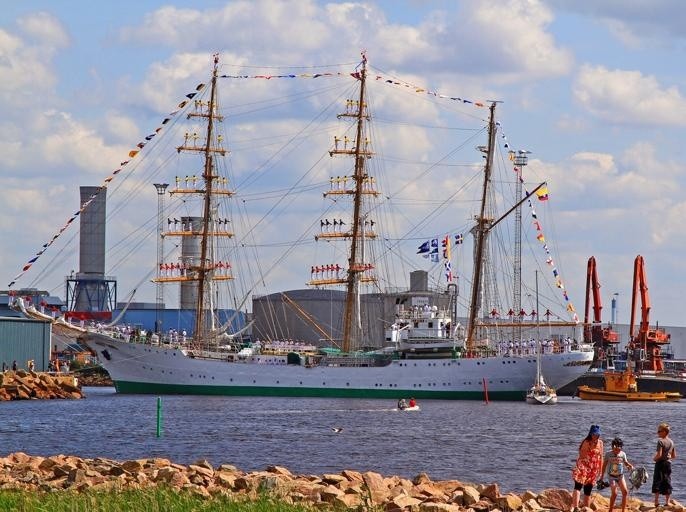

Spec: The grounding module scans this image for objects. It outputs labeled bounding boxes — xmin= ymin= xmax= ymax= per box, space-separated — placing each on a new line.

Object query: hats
xmin=589 ymin=425 xmax=605 ymax=435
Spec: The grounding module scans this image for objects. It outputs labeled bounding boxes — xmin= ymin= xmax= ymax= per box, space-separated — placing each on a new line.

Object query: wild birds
xmin=330 ymin=427 xmax=345 ymax=433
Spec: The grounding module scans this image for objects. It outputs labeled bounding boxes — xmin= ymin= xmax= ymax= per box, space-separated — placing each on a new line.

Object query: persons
xmin=255 ymin=337 xmax=311 ymax=352
xmin=47 ymin=359 xmax=70 ymax=372
xmin=498 ymin=337 xmax=572 ymax=355
xmin=11 ymin=360 xmax=17 ymax=373
xmin=28 ymin=360 xmax=35 ymax=371
xmin=7 ymin=290 xmax=86 ymax=328
xmin=90 ymin=318 xmax=186 ymax=345
xmin=530 ymin=384 xmax=551 ymax=396
xmin=599 ymin=436 xmax=634 ymax=512
xmin=571 ymin=424 xmax=602 ymax=507
xmin=408 ymin=397 xmax=415 ymax=407
xmin=400 ymin=399 xmax=408 ymax=408
xmin=211 ymin=133 xmax=233 ymax=278
xmin=489 ymin=309 xmax=501 ymax=324
xmin=310 ymin=135 xmax=377 ymax=282
xmin=651 ymin=423 xmax=676 ymax=507
xmin=407 ymin=302 xmax=431 ymax=318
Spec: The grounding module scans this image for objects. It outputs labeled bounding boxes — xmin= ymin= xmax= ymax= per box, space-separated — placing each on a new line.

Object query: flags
xmin=6 ymin=82 xmax=206 ymax=289
xmin=495 ymin=122 xmax=582 ymax=327
xmin=213 ymin=72 xmax=493 ymax=111
xmin=417 ymin=233 xmax=464 ymax=282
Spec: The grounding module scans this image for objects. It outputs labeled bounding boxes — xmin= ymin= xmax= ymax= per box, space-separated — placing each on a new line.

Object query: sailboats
xmin=7 ymin=50 xmax=602 ymax=403
xmin=525 ymin=266 xmax=560 ymax=406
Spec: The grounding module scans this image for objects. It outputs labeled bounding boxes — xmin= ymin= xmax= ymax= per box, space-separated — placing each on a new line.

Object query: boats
xmin=576 ymin=350 xmax=682 ymax=405
xmin=403 ymin=405 xmax=423 ymax=413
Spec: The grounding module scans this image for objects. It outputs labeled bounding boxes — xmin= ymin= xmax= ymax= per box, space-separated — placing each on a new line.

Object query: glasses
xmin=658 ymin=430 xmax=664 ymax=433
xmin=613 ymin=445 xmax=622 ymax=450
